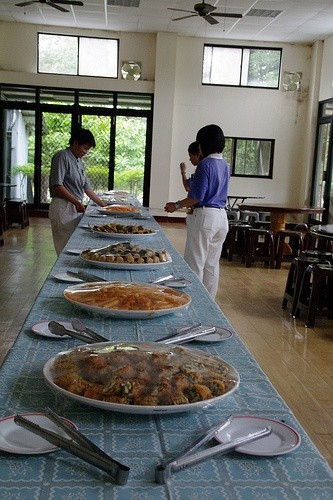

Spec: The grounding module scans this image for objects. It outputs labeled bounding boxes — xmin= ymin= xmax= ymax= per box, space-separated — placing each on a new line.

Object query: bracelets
xmin=182 ymin=171 xmax=186 ymax=174
xmin=175 ymin=201 xmax=182 ymax=209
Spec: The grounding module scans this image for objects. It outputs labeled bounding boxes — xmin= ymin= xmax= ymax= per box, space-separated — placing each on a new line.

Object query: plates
xmin=0 ymin=412 xmax=79 ymax=455
xmin=66 ymin=247 xmax=93 ymax=254
xmin=97 ymin=208 xmax=142 ymax=216
xmin=31 ymin=319 xmax=86 ymax=338
xmin=42 ymin=340 xmax=241 ymax=416
xmin=161 ymin=279 xmax=192 ymax=288
xmin=94 ymin=227 xmax=157 ymax=236
xmin=178 ymin=325 xmax=233 ymax=341
xmin=213 ymin=416 xmax=301 ymax=457
xmin=81 ymin=224 xmax=96 ymax=228
xmin=64 ymin=280 xmax=191 ymax=320
xmin=53 ymin=272 xmax=85 ymax=282
xmin=79 ymin=252 xmax=173 ymax=270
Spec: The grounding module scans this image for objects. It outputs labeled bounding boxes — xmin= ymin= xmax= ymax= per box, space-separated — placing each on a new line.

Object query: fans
xmin=13 ymin=0 xmax=85 ymax=13
xmin=166 ymin=0 xmax=243 ymax=25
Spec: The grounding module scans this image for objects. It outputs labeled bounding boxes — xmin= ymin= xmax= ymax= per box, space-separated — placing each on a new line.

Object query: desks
xmin=236 ymin=202 xmax=327 ymax=262
xmin=309 ymin=224 xmax=333 ymax=319
xmin=0 ymin=183 xmax=17 ymax=231
xmin=226 ymin=195 xmax=265 ymax=212
xmin=0 ymin=192 xmax=333 ymax=500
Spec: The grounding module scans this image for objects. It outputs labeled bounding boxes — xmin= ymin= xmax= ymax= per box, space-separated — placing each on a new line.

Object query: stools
xmin=0 ymin=196 xmax=30 ymax=231
xmin=226 ymin=209 xmax=333 ymax=328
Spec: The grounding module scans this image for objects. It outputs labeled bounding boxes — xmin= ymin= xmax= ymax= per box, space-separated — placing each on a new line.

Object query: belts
xmin=195 ymin=206 xmax=215 ymax=208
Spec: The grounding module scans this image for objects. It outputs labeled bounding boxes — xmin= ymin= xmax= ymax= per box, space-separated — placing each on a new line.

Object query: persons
xmin=164 ymin=125 xmax=230 ymax=301
xmin=49 ymin=128 xmax=112 ymax=257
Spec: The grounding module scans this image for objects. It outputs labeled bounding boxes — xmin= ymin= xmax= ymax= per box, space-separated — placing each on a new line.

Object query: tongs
xmin=48 ymin=317 xmax=110 ymax=344
xmin=87 ymin=241 xmax=130 ymax=254
xmin=150 ymin=273 xmax=186 ymax=284
xmin=67 ymin=271 xmax=107 ymax=283
xmin=155 ymin=414 xmax=272 ymax=484
xmin=154 ymin=323 xmax=218 ymax=345
xmin=14 ymin=406 xmax=131 ymax=486
